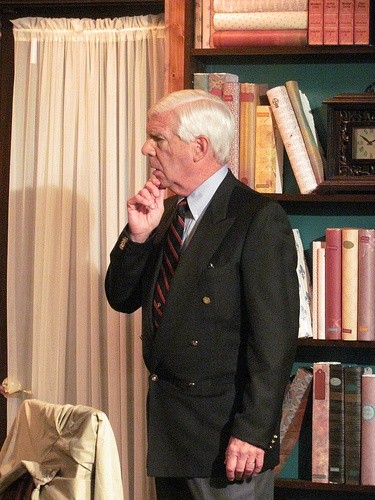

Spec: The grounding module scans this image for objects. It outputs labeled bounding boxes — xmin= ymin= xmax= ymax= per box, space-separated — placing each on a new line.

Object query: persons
xmin=105 ymin=89 xmax=300 ymax=500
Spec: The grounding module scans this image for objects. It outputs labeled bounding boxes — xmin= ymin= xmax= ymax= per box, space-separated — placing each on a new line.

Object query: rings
xmin=246 ymin=462 xmax=255 ymax=465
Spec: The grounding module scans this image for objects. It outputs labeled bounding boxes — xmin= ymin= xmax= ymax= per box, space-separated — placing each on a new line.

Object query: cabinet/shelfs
xmin=165 ymin=0 xmax=375 ymax=500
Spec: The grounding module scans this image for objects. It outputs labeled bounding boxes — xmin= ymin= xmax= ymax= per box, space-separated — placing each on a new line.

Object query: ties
xmin=153 ymin=198 xmax=188 ymax=338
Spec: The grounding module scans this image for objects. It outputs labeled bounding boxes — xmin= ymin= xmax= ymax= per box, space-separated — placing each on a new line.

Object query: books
xmin=272 ymin=360 xmax=375 ymax=486
xmin=193 ymin=70 xmax=323 ymax=195
xmin=290 ymin=226 xmax=375 ymax=342
xmin=194 ymin=1 xmax=369 ymax=49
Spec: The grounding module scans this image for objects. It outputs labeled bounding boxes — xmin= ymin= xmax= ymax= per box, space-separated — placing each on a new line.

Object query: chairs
xmin=0 ymin=399 xmax=105 ymax=500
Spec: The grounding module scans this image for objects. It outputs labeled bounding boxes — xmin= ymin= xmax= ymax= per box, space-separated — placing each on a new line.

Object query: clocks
xmin=321 ymin=81 xmax=375 ymax=194
